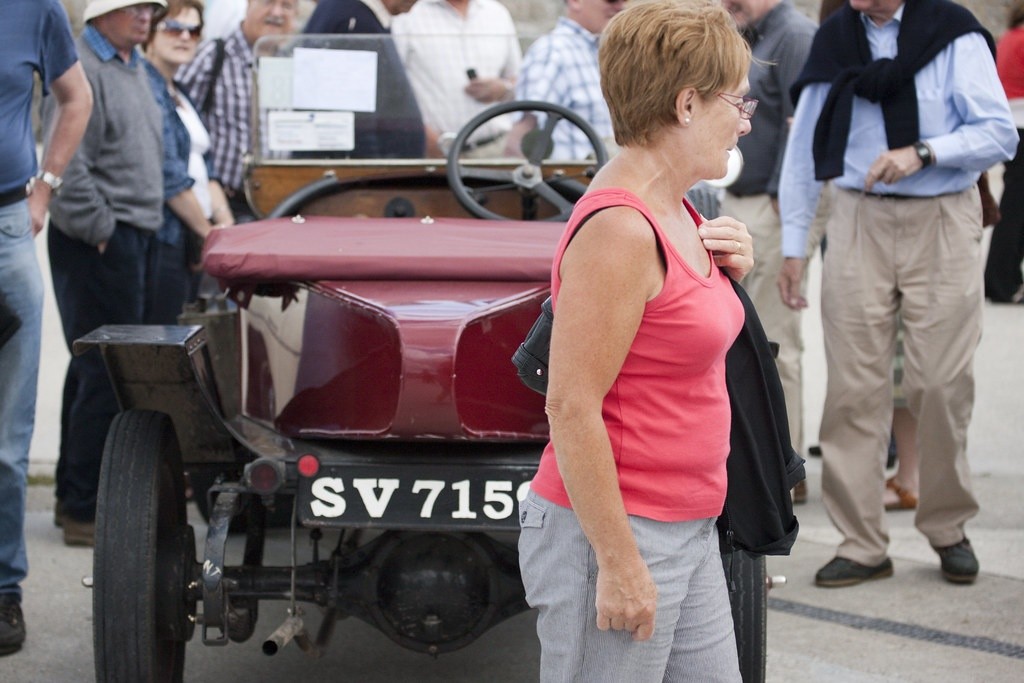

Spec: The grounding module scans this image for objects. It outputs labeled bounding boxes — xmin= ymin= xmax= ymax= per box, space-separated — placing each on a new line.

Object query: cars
xmin=71 ymin=27 xmax=787 ymax=682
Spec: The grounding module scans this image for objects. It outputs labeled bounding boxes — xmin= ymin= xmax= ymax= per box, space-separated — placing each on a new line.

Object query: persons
xmin=0 ymin=0 xmax=95 ymax=657
xmin=39 ymin=0 xmax=1024 ymax=595
xmin=516 ymin=1 xmax=754 ymax=683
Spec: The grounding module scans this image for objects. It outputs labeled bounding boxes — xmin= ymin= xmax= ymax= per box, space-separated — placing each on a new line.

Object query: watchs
xmin=35 ymin=169 xmax=65 ymax=197
xmin=913 ymin=140 xmax=933 ymax=173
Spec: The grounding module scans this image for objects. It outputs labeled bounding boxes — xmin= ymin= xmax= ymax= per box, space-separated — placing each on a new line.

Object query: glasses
xmin=156 ymin=20 xmax=202 ymax=36
xmin=718 ymin=93 xmax=759 ymax=119
xmin=117 ymin=7 xmax=155 ymax=17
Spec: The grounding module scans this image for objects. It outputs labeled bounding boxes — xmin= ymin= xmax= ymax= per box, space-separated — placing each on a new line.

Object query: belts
xmin=848 ymin=188 xmax=937 ymax=200
xmin=0 ymin=186 xmax=27 ymax=205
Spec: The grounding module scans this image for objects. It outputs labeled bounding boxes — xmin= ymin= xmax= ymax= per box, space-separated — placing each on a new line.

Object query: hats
xmin=85 ymin=0 xmax=168 ymax=22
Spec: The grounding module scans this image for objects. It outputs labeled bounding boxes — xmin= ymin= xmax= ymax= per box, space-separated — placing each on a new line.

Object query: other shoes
xmin=879 ymin=477 xmax=918 ymax=511
xmin=512 ymin=295 xmax=554 ymax=394
xmin=816 ymin=556 xmax=894 ymax=588
xmin=64 ymin=522 xmax=96 ymax=545
xmin=932 ymin=533 xmax=979 ymax=581
xmin=0 ymin=592 xmax=26 ymax=657
